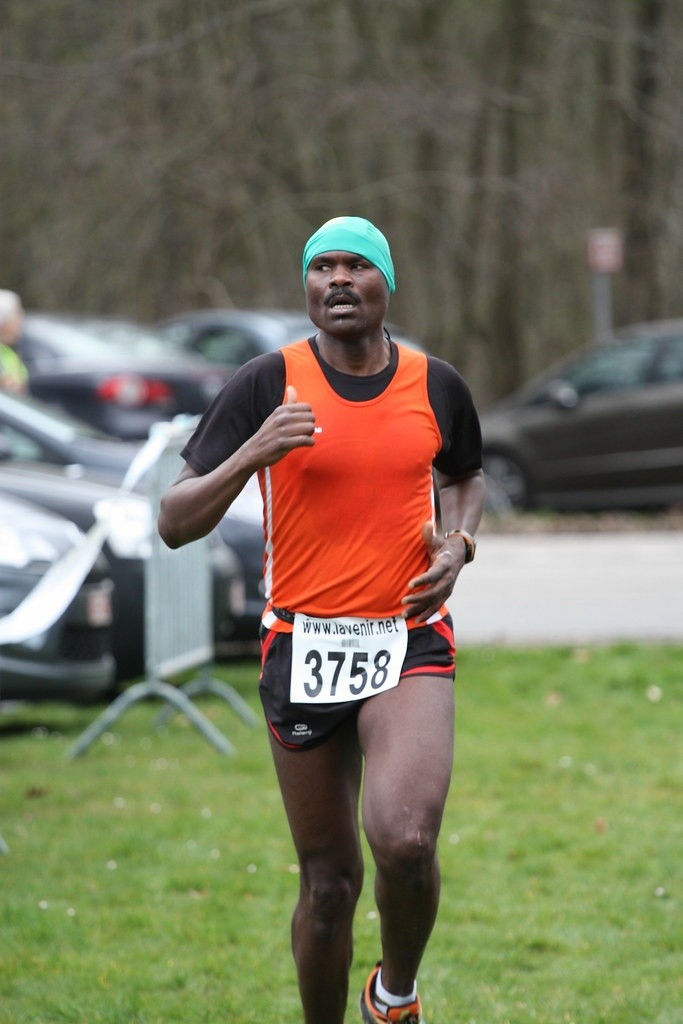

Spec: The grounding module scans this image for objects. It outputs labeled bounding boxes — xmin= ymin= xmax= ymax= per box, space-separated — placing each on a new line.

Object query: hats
xmin=302 ymin=216 xmax=394 ymax=299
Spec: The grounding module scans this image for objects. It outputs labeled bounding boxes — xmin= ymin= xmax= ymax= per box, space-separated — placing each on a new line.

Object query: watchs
xmin=444 ymin=529 xmax=476 ymax=564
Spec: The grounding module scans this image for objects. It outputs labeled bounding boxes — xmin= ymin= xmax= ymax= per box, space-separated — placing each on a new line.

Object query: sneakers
xmin=359 ymin=961 xmax=421 ymax=1024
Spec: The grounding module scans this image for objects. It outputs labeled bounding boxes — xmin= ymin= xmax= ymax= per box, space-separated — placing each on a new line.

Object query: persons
xmin=156 ymin=216 xmax=484 ymax=1024
xmin=0 ymin=290 xmax=29 ymax=398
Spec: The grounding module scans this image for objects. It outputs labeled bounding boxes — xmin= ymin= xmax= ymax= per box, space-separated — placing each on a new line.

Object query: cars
xmin=1 ymin=386 xmax=265 ymax=639
xmin=1 ymin=466 xmax=214 ymax=698
xmin=13 ymin=314 xmax=231 ymax=441
xmin=156 ymin=311 xmax=315 ymax=367
xmin=477 ymin=320 xmax=683 ymax=509
